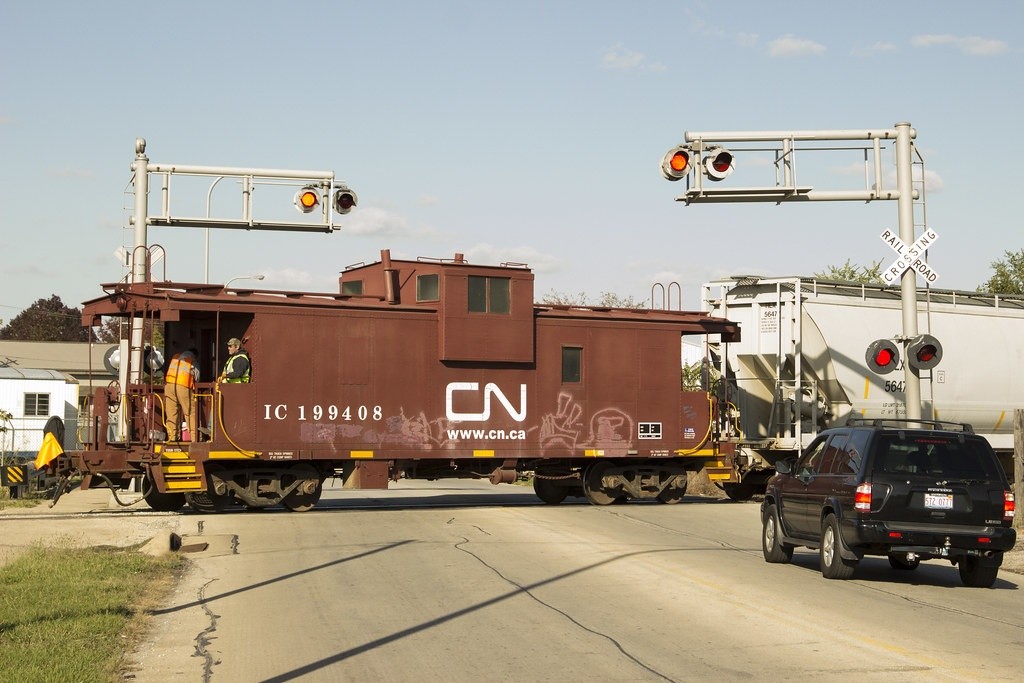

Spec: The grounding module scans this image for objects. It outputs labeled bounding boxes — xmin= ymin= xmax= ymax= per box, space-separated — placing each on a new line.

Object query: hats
xmin=227 ymin=338 xmax=242 ymax=344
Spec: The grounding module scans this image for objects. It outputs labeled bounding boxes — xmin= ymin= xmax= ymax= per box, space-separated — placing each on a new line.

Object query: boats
xmin=663 ymin=147 xmax=691 ymax=181
xmin=704 ymin=147 xmax=736 ymax=181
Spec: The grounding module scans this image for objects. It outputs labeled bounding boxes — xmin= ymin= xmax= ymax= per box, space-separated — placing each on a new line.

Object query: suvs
xmin=761 ymin=416 xmax=1018 ymax=589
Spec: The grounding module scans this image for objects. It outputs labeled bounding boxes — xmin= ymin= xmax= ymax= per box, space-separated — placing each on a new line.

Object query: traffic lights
xmin=335 ymin=192 xmax=357 ymax=215
xmin=295 ymin=188 xmax=322 ymax=213
xmin=908 ymin=336 xmax=943 ymax=370
xmin=864 ymin=340 xmax=900 ymax=375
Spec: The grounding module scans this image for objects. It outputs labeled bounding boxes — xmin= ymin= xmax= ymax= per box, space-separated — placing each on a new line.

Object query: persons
xmin=216 ymin=338 xmax=250 ymax=385
xmin=164 ymin=348 xmax=203 ymax=442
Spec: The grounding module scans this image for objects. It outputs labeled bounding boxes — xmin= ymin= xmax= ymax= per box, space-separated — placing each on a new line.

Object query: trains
xmin=0 ymin=247 xmax=1024 ymax=512
